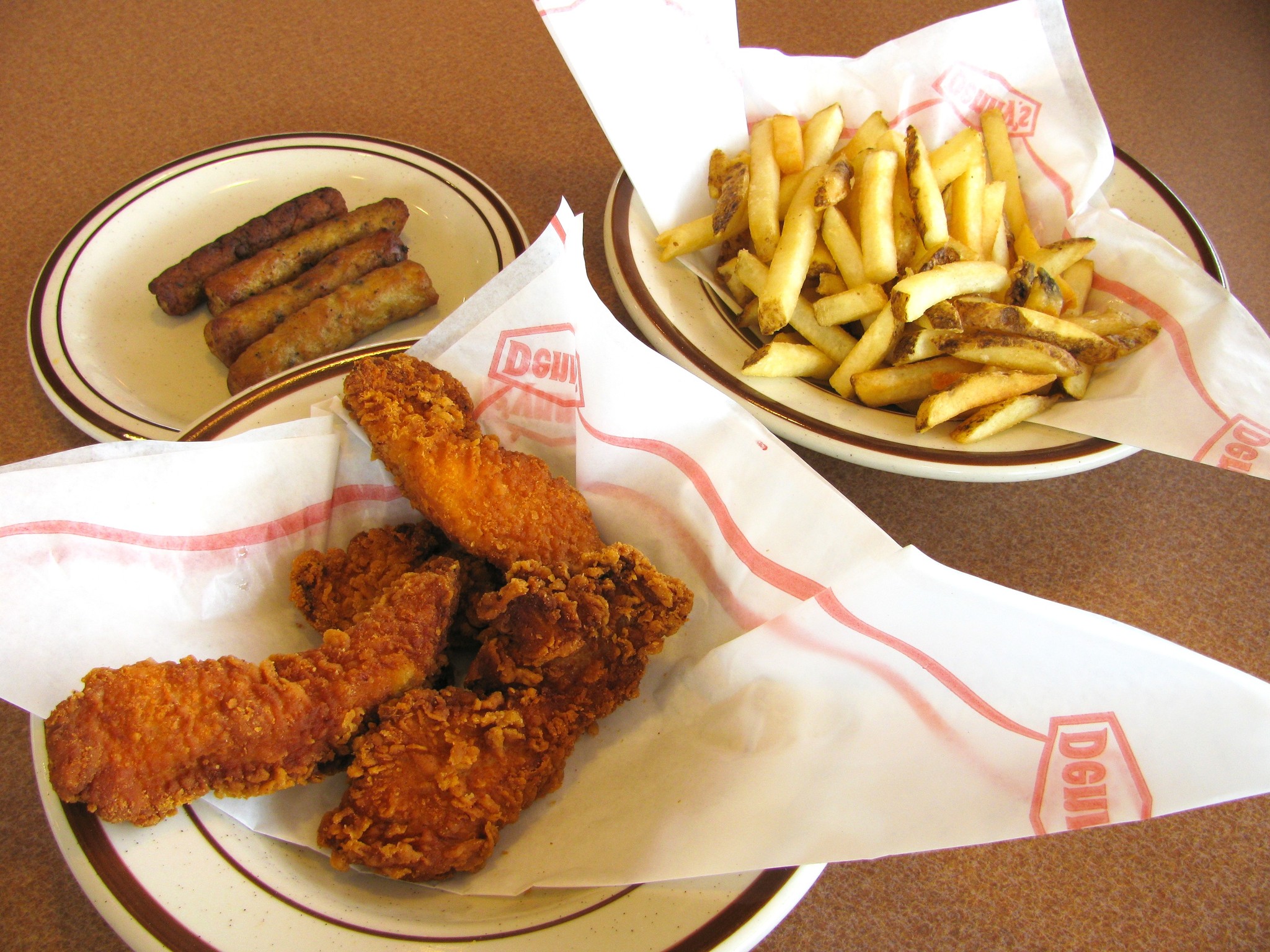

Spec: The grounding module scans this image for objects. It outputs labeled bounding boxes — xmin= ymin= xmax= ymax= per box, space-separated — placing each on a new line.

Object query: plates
xmin=31 ymin=131 xmax=529 ymax=450
xmin=597 ymin=143 xmax=1232 ymax=485
xmin=19 ymin=339 xmax=829 ymax=952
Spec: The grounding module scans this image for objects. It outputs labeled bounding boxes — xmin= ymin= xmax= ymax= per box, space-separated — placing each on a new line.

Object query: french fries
xmin=656 ymin=105 xmax=1163 ymax=446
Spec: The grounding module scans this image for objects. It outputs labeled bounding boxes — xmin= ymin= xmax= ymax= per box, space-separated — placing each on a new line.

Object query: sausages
xmin=144 ymin=183 xmax=437 ymax=397
